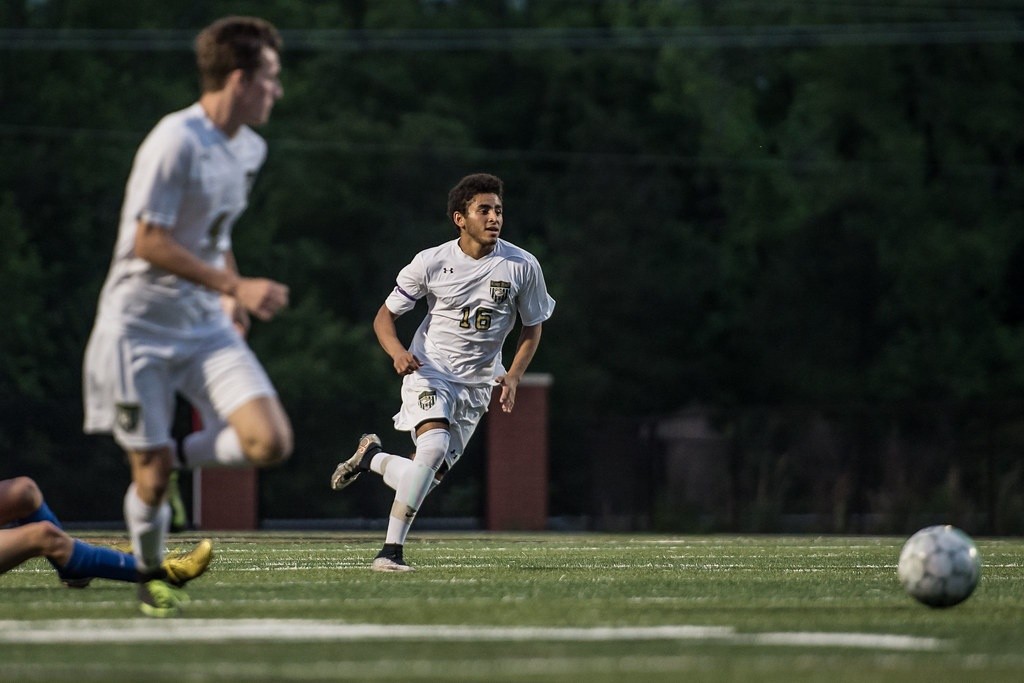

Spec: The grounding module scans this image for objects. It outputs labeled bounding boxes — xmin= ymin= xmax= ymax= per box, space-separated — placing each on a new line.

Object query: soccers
xmin=897 ymin=524 xmax=981 ymax=610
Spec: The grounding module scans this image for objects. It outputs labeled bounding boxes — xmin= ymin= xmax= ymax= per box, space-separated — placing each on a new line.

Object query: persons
xmin=0 ymin=475 xmax=215 ymax=590
xmin=79 ymin=12 xmax=294 ymax=617
xmin=330 ymin=173 xmax=555 ymax=570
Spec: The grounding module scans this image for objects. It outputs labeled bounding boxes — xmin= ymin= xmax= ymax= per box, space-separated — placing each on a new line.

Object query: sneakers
xmin=168 ymin=471 xmax=189 ymax=534
xmin=331 ymin=434 xmax=381 ymax=491
xmin=137 ymin=570 xmax=178 ymax=616
xmin=371 ymin=543 xmax=416 ymax=573
xmin=163 ymin=541 xmax=213 ymax=588
